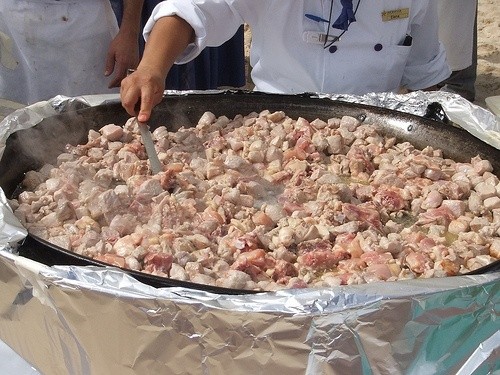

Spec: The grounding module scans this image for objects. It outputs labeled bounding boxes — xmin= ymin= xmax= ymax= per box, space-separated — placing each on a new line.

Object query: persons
xmin=120 ymin=0 xmax=452 ymax=121
xmin=432 ymin=0 xmax=477 ymax=103
xmin=1 ymin=0 xmax=144 ymax=105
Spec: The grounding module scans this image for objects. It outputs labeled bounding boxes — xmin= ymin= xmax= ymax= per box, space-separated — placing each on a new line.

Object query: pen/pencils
xmin=304 ymin=13 xmax=330 ymax=24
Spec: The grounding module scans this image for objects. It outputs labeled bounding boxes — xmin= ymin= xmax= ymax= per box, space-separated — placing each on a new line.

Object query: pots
xmin=0 ymin=93 xmax=500 ymax=297
